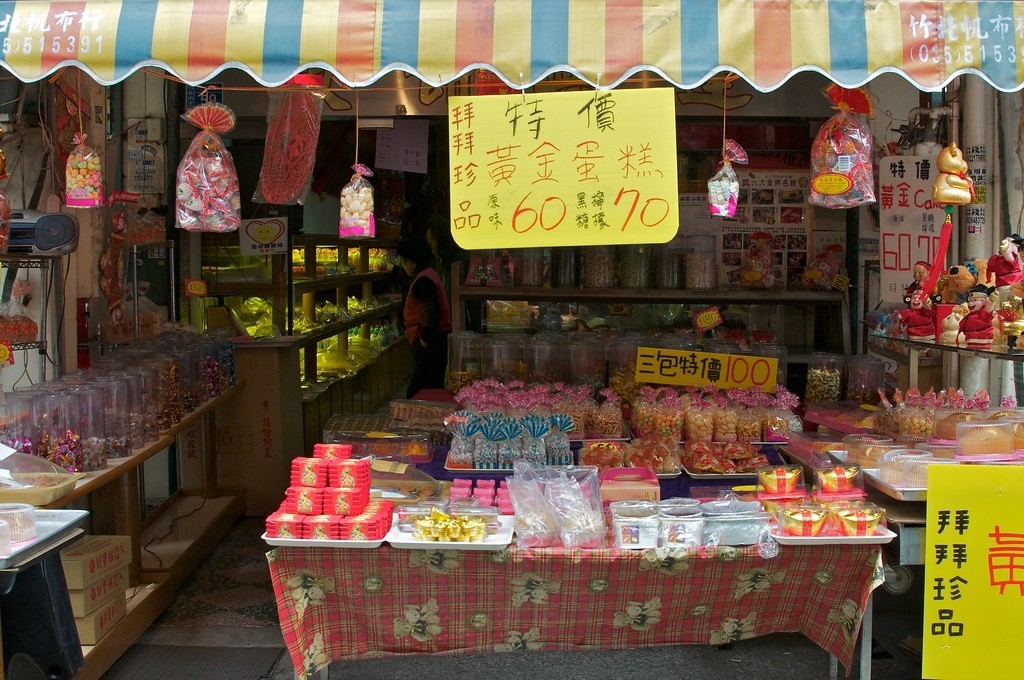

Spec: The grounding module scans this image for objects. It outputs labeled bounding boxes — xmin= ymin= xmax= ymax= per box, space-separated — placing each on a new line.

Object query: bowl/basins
xmin=705 ymin=514 xmax=772 ymax=547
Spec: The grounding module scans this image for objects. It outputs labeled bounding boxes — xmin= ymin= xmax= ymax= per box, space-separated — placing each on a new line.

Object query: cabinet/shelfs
xmin=202 ymin=233 xmax=415 ymax=496
xmin=0 ymin=257 xmax=249 ymax=680
xmin=450 ymin=259 xmax=852 ymax=368
xmin=772 ymin=333 xmax=1024 ymax=526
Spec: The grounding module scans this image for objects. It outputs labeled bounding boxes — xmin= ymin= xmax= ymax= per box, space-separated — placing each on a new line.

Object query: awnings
xmin=0 ymin=0 xmax=1024 ymax=93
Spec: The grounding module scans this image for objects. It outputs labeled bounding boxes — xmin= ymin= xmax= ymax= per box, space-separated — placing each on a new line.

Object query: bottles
xmin=0 ymin=329 xmax=232 ymax=472
xmin=514 ymin=234 xmax=717 ymax=289
xmin=447 ymin=331 xmax=886 ymax=415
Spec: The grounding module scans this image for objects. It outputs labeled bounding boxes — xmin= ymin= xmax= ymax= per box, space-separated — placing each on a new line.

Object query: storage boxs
xmin=74 ymin=590 xmax=127 ymax=645
xmin=68 ymin=565 xmax=129 ymax=617
xmin=703 ymin=517 xmax=772 ymax=545
xmin=810 ymin=462 xmax=869 ymax=503
xmin=58 ymin=535 xmax=132 ymax=590
xmin=267 ymin=431 xmax=503 ymax=540
xmin=755 ymin=464 xmax=807 ymax=506
xmin=921 ymin=303 xmax=960 ymax=343
xmin=690 ymin=485 xmax=744 ymax=505
xmin=600 ymin=466 xmax=661 ymax=500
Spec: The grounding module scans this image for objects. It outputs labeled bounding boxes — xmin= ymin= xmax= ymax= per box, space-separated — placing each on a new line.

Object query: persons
xmin=906 ymin=261 xmax=931 ymax=294
xmin=958 ymin=284 xmax=999 ymax=347
xmin=396 ymin=239 xmax=451 ymax=399
xmin=425 ymin=218 xmax=480 ymax=331
xmin=898 ymin=286 xmax=935 ymax=340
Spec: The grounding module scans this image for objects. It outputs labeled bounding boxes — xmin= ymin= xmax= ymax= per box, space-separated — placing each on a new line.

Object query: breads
xmin=846 ymin=411 xmax=1024 ymax=487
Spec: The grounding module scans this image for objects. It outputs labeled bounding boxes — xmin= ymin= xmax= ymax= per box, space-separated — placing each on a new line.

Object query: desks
xmin=276 ymin=527 xmax=882 ymax=680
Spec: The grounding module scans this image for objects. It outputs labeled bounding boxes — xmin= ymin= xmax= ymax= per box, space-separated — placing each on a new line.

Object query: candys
xmin=0 ymin=342 xmax=236 ymax=473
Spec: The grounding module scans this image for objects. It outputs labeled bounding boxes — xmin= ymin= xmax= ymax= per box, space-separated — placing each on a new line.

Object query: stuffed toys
xmin=948 ymin=257 xmax=995 ymax=295
